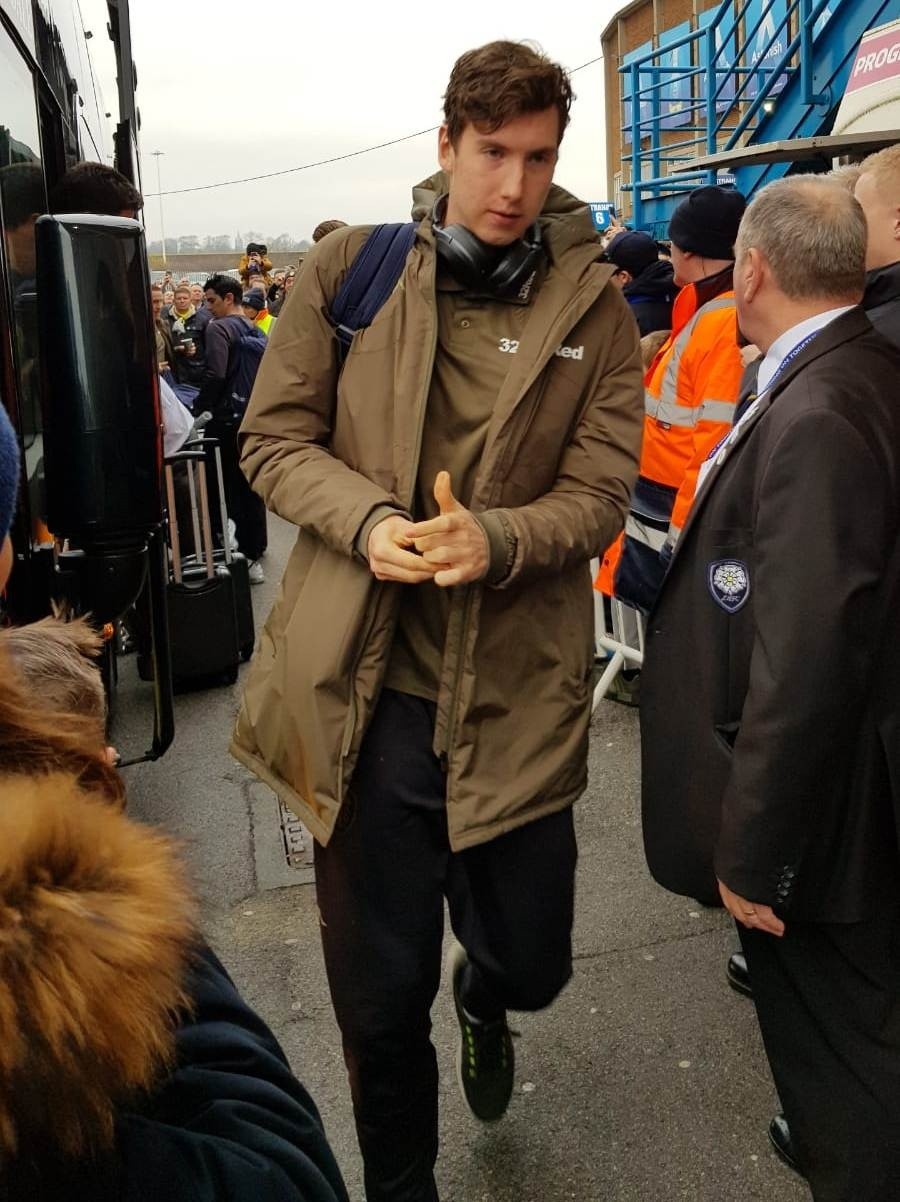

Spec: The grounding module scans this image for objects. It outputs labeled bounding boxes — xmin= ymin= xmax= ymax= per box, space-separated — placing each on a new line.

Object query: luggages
xmin=168 ymin=438 xmax=255 ymax=664
xmin=162 ymin=452 xmax=239 ymax=694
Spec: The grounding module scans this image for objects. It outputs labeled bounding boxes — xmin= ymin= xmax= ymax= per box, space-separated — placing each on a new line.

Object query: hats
xmin=0 ymin=403 xmax=21 ymax=544
xmin=667 ymin=186 xmax=747 ymax=260
xmin=606 ymin=229 xmax=659 ymax=263
xmin=242 ymin=287 xmax=266 ymax=310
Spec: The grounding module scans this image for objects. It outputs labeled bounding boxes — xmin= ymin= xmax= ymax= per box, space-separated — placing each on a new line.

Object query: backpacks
xmin=219 ymin=316 xmax=269 ymax=429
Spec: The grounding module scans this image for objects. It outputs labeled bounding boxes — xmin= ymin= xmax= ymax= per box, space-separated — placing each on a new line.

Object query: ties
xmin=733 ymin=374 xmax=759 ymax=426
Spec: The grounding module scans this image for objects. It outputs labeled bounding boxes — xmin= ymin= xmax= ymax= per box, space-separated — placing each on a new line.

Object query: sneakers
xmin=450 ymin=941 xmax=521 ymax=1124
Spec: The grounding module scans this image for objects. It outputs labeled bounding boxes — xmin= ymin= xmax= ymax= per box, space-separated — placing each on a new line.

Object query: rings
xmin=744 ymin=910 xmax=755 ymax=915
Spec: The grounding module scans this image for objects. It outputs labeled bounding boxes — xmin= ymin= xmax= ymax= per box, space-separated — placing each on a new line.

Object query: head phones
xmin=430 ymin=192 xmax=544 ymax=298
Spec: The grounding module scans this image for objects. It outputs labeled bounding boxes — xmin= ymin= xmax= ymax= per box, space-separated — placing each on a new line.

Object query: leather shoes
xmin=726 ymin=951 xmax=754 ymax=997
xmin=770 ymin=1113 xmax=801 ymax=1171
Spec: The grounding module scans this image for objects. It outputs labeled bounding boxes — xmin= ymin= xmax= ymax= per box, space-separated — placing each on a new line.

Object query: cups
xmin=180 ymin=337 xmax=192 ymax=352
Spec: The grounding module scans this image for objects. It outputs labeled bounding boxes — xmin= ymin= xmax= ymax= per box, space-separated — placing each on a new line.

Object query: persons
xmin=596 ymin=211 xmax=678 ymax=373
xmin=636 ymin=174 xmax=900 ymax=1202
xmin=65 ymin=161 xmax=144 ymax=219
xmin=850 ymin=142 xmax=900 ymax=345
xmin=227 ymin=40 xmax=647 ymax=1202
xmin=237 ymin=243 xmax=297 ymax=336
xmin=1 ymin=161 xmax=50 ymax=361
xmin=0 ymin=402 xmax=351 ymax=1202
xmin=193 ymin=275 xmax=269 ymax=585
xmin=313 ymin=220 xmax=350 ymax=242
xmin=592 ymin=183 xmax=751 ymax=619
xmin=151 ymin=270 xmax=213 ymax=409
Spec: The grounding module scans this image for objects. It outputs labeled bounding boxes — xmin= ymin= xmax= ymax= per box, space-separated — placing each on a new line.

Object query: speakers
xmin=35 ymin=210 xmax=162 ymax=549
xmin=157 ymin=564 xmax=240 ymax=695
